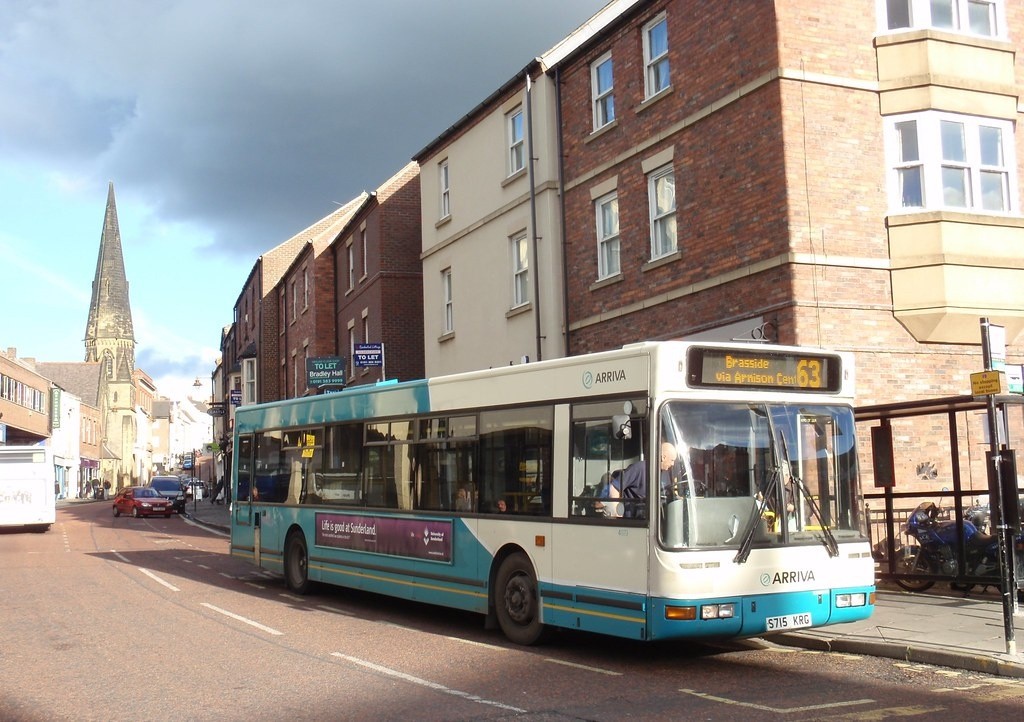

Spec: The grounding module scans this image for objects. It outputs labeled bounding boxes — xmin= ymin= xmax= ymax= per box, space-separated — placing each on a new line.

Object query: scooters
xmin=950 ymin=500 xmax=1024 ymax=593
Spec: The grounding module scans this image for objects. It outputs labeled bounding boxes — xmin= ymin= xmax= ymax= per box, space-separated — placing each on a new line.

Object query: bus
xmin=229 ymin=340 xmax=877 ymax=647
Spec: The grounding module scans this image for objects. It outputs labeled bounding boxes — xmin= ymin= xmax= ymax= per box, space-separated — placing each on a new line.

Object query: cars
xmin=178 ymin=474 xmax=197 ymax=486
xmin=112 ymin=487 xmax=174 ymax=519
xmin=147 ymin=476 xmax=187 ymax=514
xmin=182 ymin=455 xmax=192 ymax=469
xmin=187 ymin=480 xmax=209 ymax=498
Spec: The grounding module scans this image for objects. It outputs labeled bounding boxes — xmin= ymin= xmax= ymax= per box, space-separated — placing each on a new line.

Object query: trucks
xmin=1 ymin=446 xmax=60 ymax=532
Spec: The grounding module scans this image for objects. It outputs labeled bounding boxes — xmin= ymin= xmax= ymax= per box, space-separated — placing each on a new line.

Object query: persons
xmin=455 ymin=489 xmax=471 ymax=511
xmin=104 ymin=480 xmax=111 ymax=500
xmin=497 ymin=500 xmax=507 ymax=513
xmin=246 ymin=487 xmax=263 ymax=501
xmin=590 ymin=442 xmax=677 ymax=519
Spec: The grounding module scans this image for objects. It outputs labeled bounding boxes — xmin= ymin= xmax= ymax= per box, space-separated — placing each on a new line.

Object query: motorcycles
xmin=891 ymin=502 xmax=1024 ymax=603
